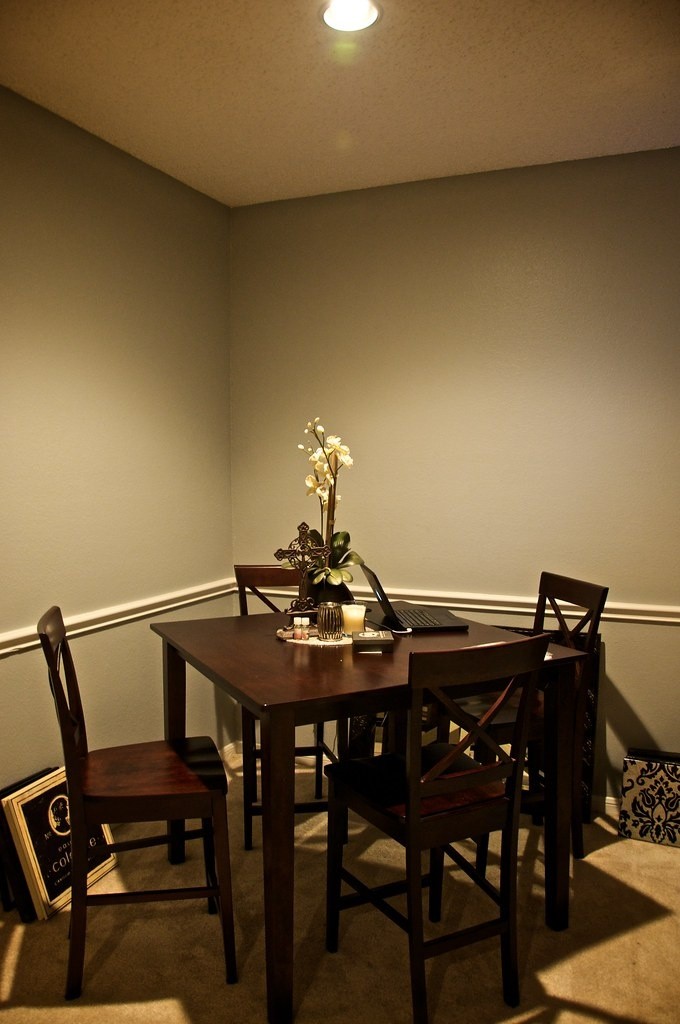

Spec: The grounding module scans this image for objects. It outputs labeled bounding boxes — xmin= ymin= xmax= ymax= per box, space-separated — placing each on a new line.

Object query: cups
xmin=340 ymin=601 xmax=368 ymax=639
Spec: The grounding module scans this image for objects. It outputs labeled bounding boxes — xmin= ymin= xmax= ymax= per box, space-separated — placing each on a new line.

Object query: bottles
xmin=302 ymin=618 xmax=309 ymax=640
xmin=294 ymin=617 xmax=302 ymax=638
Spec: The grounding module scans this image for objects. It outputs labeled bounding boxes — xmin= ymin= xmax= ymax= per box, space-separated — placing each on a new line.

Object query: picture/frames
xmin=0 ymin=765 xmax=120 ymax=925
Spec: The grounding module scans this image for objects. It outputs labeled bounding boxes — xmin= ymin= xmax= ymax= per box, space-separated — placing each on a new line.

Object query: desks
xmin=150 ymin=601 xmax=587 ymax=1024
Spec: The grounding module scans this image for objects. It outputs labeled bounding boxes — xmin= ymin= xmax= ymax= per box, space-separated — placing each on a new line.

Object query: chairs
xmin=36 ymin=606 xmax=238 ymax=998
xmin=322 ymin=629 xmax=553 ymax=1024
xmin=234 ymin=563 xmax=342 ymax=851
xmin=435 ymin=571 xmax=610 ymax=890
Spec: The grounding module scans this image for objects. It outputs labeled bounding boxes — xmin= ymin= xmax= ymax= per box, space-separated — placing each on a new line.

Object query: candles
xmin=342 ymin=604 xmax=365 ymax=637
xmin=317 ymin=601 xmax=343 ymax=642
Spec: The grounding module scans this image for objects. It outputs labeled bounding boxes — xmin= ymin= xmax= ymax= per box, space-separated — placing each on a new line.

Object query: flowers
xmin=298 ymin=416 xmax=403 ymax=628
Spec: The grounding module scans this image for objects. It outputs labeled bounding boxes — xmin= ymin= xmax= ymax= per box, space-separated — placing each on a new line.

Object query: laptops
xmin=360 ymin=563 xmax=469 ymax=633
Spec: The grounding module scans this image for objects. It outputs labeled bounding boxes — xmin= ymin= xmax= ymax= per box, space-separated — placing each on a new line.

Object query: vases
xmin=302 ymin=571 xmax=356 ymax=623
